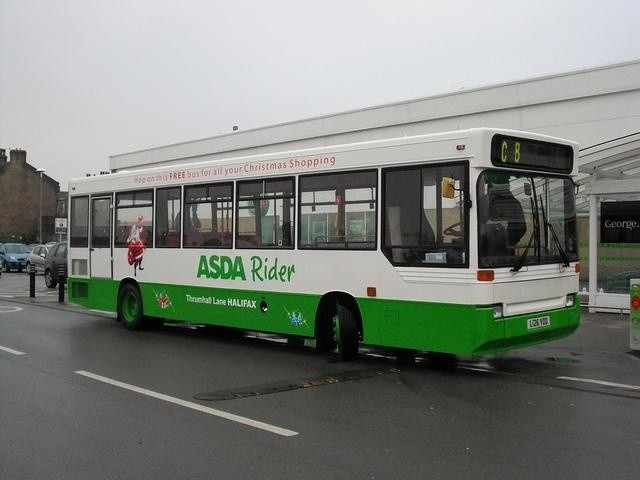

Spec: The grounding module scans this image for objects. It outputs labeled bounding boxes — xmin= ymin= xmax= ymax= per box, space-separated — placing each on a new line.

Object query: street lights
xmin=36 ymin=169 xmax=46 ymax=246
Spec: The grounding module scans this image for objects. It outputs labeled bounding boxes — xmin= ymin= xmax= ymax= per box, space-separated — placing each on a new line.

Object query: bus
xmin=66 ymin=127 xmax=584 ymax=365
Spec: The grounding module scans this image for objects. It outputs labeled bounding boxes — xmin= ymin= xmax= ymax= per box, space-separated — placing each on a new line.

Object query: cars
xmin=0 ymin=240 xmax=65 ymax=289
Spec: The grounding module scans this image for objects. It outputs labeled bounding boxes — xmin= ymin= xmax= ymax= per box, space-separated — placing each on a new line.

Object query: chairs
xmin=118 ymin=227 xmax=260 ymax=249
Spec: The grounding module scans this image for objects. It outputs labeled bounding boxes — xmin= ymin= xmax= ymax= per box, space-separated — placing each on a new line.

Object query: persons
xmin=480 ymin=173 xmax=528 ymax=255
